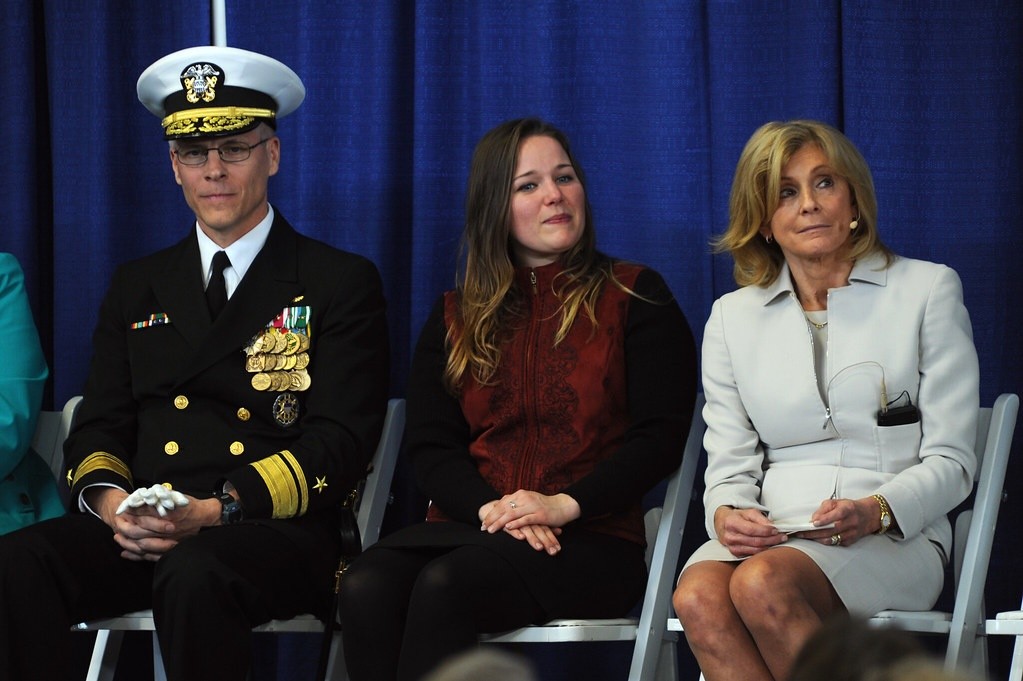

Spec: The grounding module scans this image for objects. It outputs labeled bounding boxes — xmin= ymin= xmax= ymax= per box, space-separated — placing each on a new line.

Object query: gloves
xmin=115 ymin=484 xmax=190 ymax=519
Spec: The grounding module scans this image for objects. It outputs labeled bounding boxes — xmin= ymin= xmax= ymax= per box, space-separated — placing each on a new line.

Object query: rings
xmin=510 ymin=502 xmax=517 ymax=509
xmin=831 ymin=534 xmax=841 ymax=545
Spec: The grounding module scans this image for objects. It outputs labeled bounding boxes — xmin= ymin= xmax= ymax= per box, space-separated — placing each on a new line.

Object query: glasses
xmin=173 ymin=138 xmax=270 ymax=165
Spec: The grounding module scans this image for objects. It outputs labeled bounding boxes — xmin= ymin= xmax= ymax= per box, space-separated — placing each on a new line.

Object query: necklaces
xmin=808 ymin=318 xmax=827 ymax=328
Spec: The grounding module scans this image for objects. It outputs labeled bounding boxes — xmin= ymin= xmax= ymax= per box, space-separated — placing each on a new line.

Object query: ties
xmin=204 ymin=251 xmax=232 ymax=323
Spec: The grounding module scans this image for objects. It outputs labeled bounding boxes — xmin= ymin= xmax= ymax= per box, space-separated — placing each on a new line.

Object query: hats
xmin=136 ymin=45 xmax=307 ymax=141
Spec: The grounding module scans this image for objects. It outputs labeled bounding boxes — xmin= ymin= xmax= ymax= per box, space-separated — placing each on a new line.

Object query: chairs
xmin=33 ymin=395 xmax=85 ymax=482
xmin=322 ymin=400 xmax=638 ymax=681
xmin=71 ymin=603 xmax=322 ymax=681
xmin=625 ymin=394 xmax=1019 ymax=681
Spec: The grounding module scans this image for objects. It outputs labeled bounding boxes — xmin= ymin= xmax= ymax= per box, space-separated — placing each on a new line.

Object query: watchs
xmin=873 ymin=494 xmax=891 ymax=535
xmin=214 ymin=493 xmax=242 ymax=526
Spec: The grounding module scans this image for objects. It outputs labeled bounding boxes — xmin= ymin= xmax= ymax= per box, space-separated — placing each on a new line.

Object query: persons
xmin=0 ymin=46 xmax=388 ymax=680
xmin=342 ymin=117 xmax=699 ymax=681
xmin=432 ymin=648 xmax=532 ymax=681
xmin=789 ymin=615 xmax=957 ymax=681
xmin=0 ymin=253 xmax=66 ymax=551
xmin=673 ymin=120 xmax=980 ymax=681
